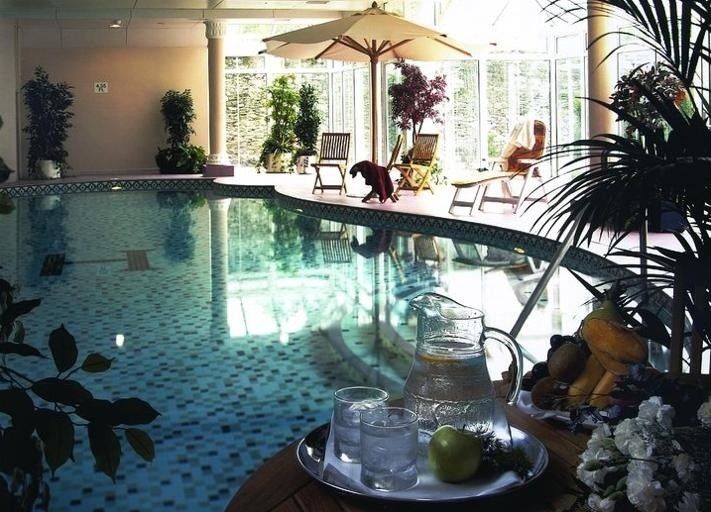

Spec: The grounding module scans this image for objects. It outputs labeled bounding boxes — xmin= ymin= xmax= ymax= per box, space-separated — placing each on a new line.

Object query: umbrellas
xmin=260 ymin=1 xmax=474 ymax=199
xmin=307 ymin=225 xmax=418 ymax=401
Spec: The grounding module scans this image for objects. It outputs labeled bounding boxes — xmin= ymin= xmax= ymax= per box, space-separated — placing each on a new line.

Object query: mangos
xmin=583 ymin=319 xmax=646 ymax=375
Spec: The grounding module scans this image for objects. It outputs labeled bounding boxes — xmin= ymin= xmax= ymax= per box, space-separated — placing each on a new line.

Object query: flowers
xmin=567 ymin=391 xmax=706 ymax=510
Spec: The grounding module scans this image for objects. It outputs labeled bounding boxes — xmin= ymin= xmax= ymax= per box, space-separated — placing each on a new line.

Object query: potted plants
xmin=17 ymin=64 xmax=78 ymax=180
xmin=153 ymin=88 xmax=208 ymax=172
xmin=254 ymin=72 xmax=323 ymax=174
xmin=384 ymin=61 xmax=451 ymax=190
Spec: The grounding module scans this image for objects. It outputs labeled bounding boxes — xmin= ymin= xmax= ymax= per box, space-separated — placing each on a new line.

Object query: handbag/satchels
xmin=502 ymin=143 xmax=532 ymax=170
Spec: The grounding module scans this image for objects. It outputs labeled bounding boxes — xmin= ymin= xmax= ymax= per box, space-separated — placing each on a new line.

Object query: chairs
xmin=306 ymin=119 xmax=551 ymax=219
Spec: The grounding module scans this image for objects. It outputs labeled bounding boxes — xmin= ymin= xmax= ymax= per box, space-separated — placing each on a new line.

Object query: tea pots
xmin=405 ymin=293 xmax=522 ymax=456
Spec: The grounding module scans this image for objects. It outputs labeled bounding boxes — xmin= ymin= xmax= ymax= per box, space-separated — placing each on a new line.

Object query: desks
xmin=224 ymin=374 xmax=710 ymax=511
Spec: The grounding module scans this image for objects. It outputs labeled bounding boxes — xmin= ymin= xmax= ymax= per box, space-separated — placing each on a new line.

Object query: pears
xmin=583 ymin=290 xmax=624 ymax=328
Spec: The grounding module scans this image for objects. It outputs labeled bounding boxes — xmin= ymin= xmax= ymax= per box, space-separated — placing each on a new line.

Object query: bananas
xmin=567 ymin=352 xmax=619 ymax=408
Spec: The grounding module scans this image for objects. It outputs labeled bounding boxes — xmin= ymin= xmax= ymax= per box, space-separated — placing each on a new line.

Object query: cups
xmin=361 ymin=407 xmax=422 ymax=492
xmin=333 ymin=387 xmax=389 ymax=462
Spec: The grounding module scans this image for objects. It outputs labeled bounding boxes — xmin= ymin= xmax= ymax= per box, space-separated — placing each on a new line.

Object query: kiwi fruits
xmin=530 ymin=376 xmax=564 ymax=409
xmin=547 ymin=344 xmax=586 ymax=381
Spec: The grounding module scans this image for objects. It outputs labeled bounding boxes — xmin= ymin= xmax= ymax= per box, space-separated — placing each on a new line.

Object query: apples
xmin=427 ymin=423 xmax=481 ymax=484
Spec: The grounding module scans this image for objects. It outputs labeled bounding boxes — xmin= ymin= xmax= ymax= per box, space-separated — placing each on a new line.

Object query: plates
xmin=297 ymin=417 xmax=551 ymax=502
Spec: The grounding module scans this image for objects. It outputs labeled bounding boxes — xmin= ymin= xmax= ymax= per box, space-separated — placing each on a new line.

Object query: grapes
xmin=521 ymin=335 xmax=576 ymax=389
